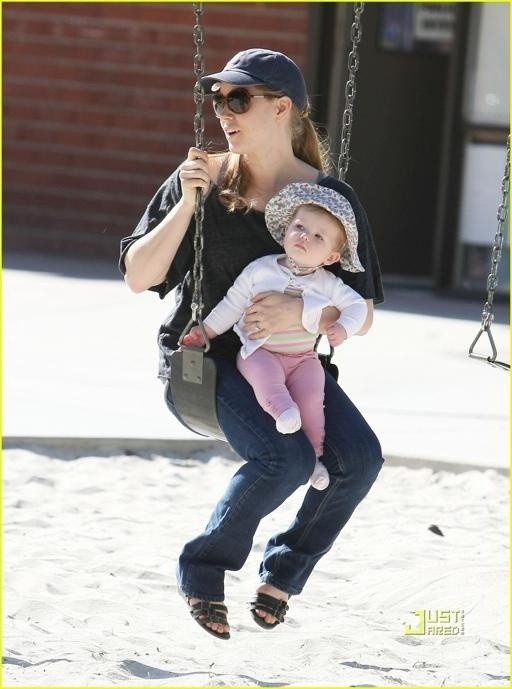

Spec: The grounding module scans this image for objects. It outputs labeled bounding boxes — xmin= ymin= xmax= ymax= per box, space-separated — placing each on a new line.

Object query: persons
xmin=181 ymin=183 xmax=367 ymax=492
xmin=117 ymin=47 xmax=384 ymax=640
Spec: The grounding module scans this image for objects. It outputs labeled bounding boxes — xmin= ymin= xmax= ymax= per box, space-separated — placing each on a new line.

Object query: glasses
xmin=212 ymin=85 xmax=284 ymax=114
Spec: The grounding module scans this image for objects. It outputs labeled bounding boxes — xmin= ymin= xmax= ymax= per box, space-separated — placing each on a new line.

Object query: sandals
xmin=248 ymin=581 xmax=291 ymax=629
xmin=180 ymin=584 xmax=231 ymax=640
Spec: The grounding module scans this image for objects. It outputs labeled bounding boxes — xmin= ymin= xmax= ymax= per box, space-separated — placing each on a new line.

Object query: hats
xmin=200 ymin=47 xmax=307 ymax=111
xmin=264 ymin=180 xmax=366 ymax=273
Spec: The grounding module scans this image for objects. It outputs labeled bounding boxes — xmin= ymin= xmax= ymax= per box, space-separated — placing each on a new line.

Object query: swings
xmin=468 ymin=133 xmax=511 ymax=375
xmin=170 ymin=1 xmax=365 ymax=443
